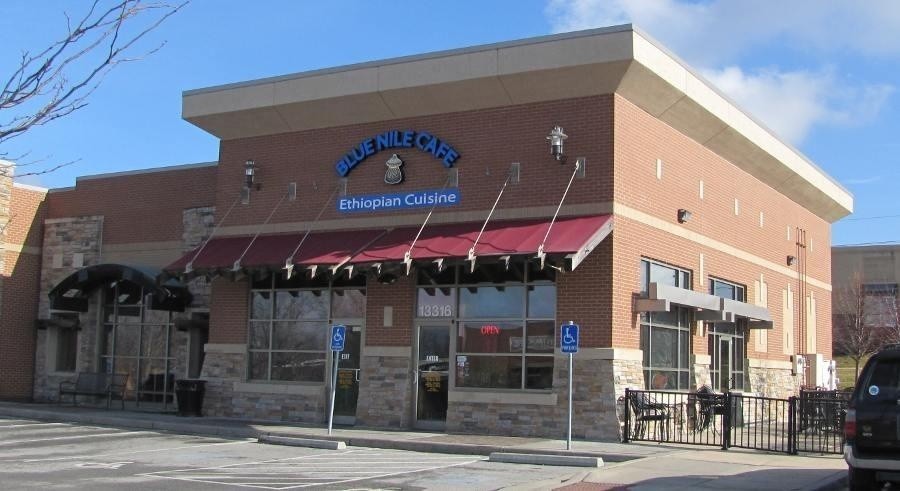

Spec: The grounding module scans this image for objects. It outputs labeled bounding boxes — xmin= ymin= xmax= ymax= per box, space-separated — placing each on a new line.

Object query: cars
xmin=507 ymin=362 xmax=553 ymax=389
xmin=417 ymin=356 xmax=482 ymax=385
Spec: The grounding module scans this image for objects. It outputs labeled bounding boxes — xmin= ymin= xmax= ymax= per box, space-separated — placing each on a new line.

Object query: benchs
xmin=56 ymin=372 xmax=130 ymax=412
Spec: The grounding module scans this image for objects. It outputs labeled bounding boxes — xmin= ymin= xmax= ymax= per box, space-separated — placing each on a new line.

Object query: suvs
xmin=841 ymin=345 xmax=900 ymax=490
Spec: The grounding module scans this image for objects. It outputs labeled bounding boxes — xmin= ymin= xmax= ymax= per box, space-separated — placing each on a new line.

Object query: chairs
xmin=797 ymin=386 xmax=856 ymax=457
xmin=627 ymin=387 xmax=728 ymax=442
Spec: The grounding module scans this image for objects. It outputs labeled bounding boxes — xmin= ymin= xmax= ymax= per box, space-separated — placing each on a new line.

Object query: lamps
xmin=546 ymin=127 xmax=570 ymax=165
xmin=680 ymin=209 xmax=691 ymax=224
xmin=243 ymin=160 xmax=262 ymax=191
xmin=787 ymin=257 xmax=797 ymax=266
